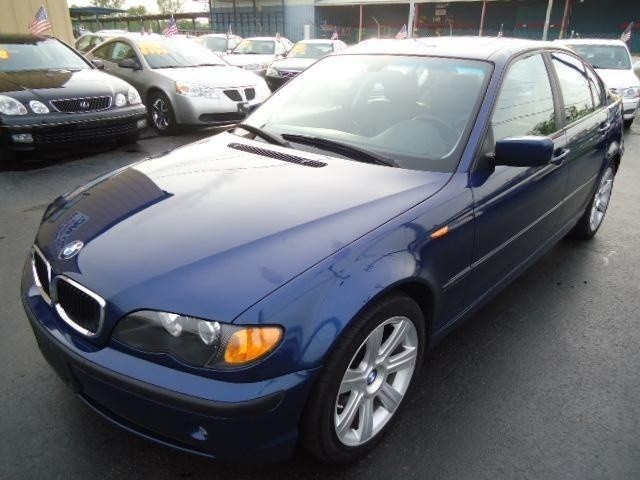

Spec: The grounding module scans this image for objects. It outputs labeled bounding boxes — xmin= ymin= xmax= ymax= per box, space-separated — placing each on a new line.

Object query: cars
xmin=74 ymin=29 xmax=131 ymax=58
xmin=266 ymin=39 xmax=348 ymax=88
xmin=222 ymin=35 xmax=295 ymax=75
xmin=552 ymin=38 xmax=639 ymax=127
xmin=199 ymin=34 xmax=246 ymax=62
xmin=85 ymin=36 xmax=273 ymax=134
xmin=0 ymin=31 xmax=148 ymax=159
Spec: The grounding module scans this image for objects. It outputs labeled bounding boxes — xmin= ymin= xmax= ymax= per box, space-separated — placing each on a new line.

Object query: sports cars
xmin=19 ymin=36 xmax=624 ymax=466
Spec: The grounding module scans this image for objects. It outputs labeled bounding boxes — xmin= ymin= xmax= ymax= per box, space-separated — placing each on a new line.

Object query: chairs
xmin=426 ymin=71 xmax=484 ymax=137
xmin=351 ymin=66 xmax=421 ymax=130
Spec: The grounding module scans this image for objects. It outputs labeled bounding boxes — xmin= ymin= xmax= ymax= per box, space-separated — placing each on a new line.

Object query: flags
xmin=622 ymin=30 xmax=631 ymax=42
xmin=331 ymin=29 xmax=338 ymax=40
xmin=396 ymin=25 xmax=407 ymax=39
xmin=28 ymin=7 xmax=48 ymax=36
xmin=163 ymin=18 xmax=179 ymax=36
